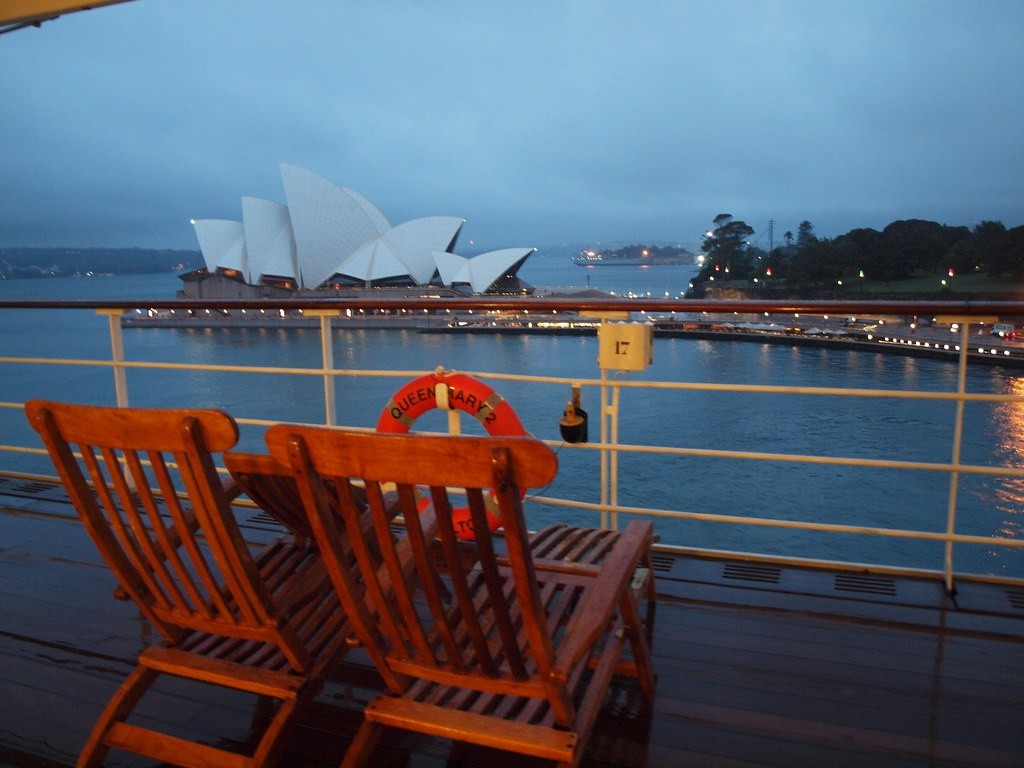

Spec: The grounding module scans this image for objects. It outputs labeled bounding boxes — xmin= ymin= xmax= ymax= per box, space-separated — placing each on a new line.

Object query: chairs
xmin=268 ymin=421 xmax=660 ymax=767
xmin=23 ymin=401 xmax=452 ymax=767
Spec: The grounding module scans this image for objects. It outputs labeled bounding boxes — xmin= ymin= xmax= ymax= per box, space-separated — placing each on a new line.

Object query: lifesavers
xmin=380 ymin=370 xmax=522 ymax=542
